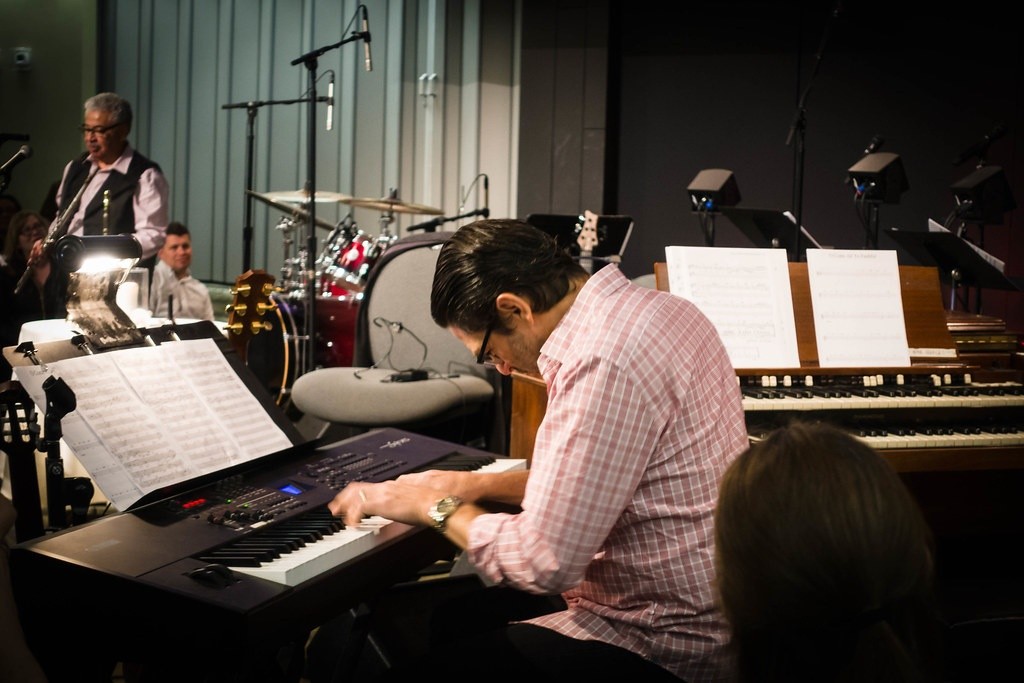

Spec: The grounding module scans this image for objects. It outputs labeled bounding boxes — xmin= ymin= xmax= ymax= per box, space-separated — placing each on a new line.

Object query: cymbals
xmin=337 ymin=194 xmax=446 ymax=216
xmin=261 ymin=188 xmax=352 ymax=206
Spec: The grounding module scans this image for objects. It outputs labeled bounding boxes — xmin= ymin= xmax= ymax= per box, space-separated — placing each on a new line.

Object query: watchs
xmin=431 ymin=495 xmax=464 ymax=531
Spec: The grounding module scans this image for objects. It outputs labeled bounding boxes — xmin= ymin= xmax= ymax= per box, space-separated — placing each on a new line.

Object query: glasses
xmin=475 ymin=303 xmax=504 ymax=370
xmin=77 ymin=122 xmax=121 ymax=138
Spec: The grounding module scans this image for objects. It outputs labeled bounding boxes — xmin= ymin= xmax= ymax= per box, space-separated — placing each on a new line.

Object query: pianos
xmin=8 ymin=423 xmax=531 ymax=683
xmin=507 ymin=259 xmax=1024 ymax=683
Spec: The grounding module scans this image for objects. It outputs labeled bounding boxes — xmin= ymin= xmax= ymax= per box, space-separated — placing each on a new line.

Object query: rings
xmin=360 ymin=493 xmax=366 ymax=499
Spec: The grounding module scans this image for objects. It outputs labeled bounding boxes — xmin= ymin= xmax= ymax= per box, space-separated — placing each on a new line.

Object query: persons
xmin=149 ymin=223 xmax=214 ymax=321
xmin=717 ymin=425 xmax=961 ymax=683
xmin=0 ymin=93 xmax=170 ymax=378
xmin=325 ymin=219 xmax=751 ymax=683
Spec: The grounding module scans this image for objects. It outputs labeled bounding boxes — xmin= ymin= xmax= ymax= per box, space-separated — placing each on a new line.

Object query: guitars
xmin=574 ymin=209 xmax=602 ymax=276
xmin=222 ymin=268 xmax=279 ymax=367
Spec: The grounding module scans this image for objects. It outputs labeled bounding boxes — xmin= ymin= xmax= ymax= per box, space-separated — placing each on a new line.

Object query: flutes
xmin=102 ymin=188 xmax=111 ymax=236
xmin=12 ymin=165 xmax=101 ymax=296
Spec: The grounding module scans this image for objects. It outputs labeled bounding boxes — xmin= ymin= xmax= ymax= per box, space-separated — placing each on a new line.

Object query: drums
xmin=309 ymin=228 xmax=392 ymax=368
xmin=247 ymin=286 xmax=311 ymax=422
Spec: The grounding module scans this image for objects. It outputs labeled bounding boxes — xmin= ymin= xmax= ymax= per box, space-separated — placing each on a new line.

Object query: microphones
xmin=845 ymin=134 xmax=884 ymax=184
xmin=484 ymin=175 xmax=489 ymax=220
xmin=363 ymin=6 xmax=372 ymax=71
xmin=953 ymin=121 xmax=1008 ymax=165
xmin=0 ymin=144 xmax=33 ymax=173
xmin=325 ymin=73 xmax=335 ymax=129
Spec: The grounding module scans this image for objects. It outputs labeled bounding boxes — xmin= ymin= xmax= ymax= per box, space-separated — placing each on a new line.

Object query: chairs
xmin=290 ymin=232 xmax=504 ymax=458
xmin=524 ymin=211 xmax=635 ymax=272
